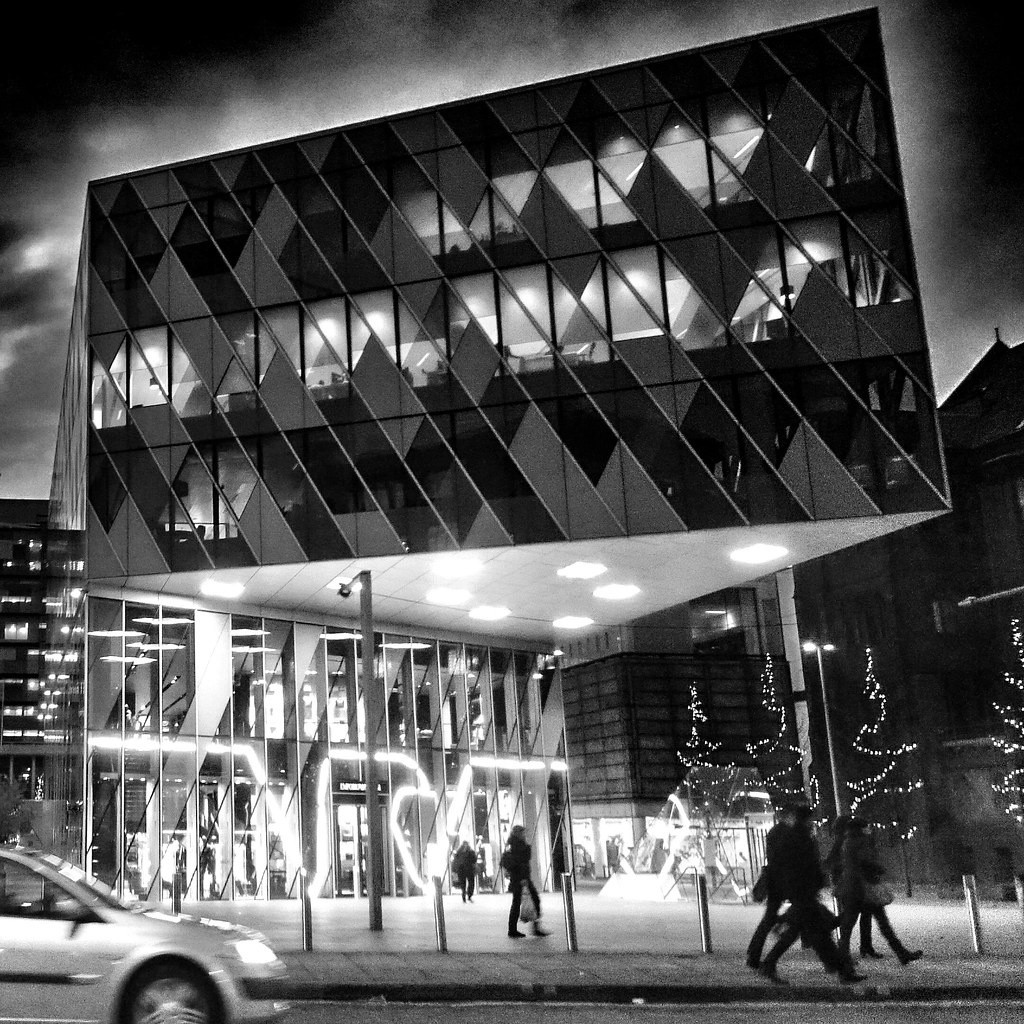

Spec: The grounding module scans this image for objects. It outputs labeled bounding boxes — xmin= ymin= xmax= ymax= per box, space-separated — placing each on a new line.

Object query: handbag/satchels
xmin=862 ymin=880 xmax=895 ymax=908
xmin=519 ymin=886 xmax=537 ymax=923
xmin=754 ymin=865 xmax=769 ymax=903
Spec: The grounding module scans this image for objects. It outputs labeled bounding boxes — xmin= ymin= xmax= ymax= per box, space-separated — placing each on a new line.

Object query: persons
xmin=503 ymin=825 xmax=550 ymax=938
xmin=828 ymin=815 xmax=924 ymax=966
xmin=455 ymin=841 xmax=477 ymax=903
xmin=746 ymin=802 xmax=869 ymax=985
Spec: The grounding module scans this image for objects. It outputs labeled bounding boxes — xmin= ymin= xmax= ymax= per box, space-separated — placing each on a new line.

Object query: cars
xmin=0 ymin=841 xmax=290 ymax=1024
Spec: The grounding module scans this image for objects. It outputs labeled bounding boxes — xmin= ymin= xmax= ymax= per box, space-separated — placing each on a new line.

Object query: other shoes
xmin=507 ymin=930 xmax=525 ymax=938
xmin=759 ymin=963 xmax=790 ymax=986
xmin=899 ymin=950 xmax=923 ymax=966
xmin=840 ymin=970 xmax=868 ymax=985
xmin=745 ymin=955 xmax=761 ymax=971
xmin=861 ymin=945 xmax=885 ymax=960
xmin=534 ymin=930 xmax=547 ymax=936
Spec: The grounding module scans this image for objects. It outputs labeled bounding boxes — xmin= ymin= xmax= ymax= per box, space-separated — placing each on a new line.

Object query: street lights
xmin=802 ymin=639 xmax=844 ymax=817
